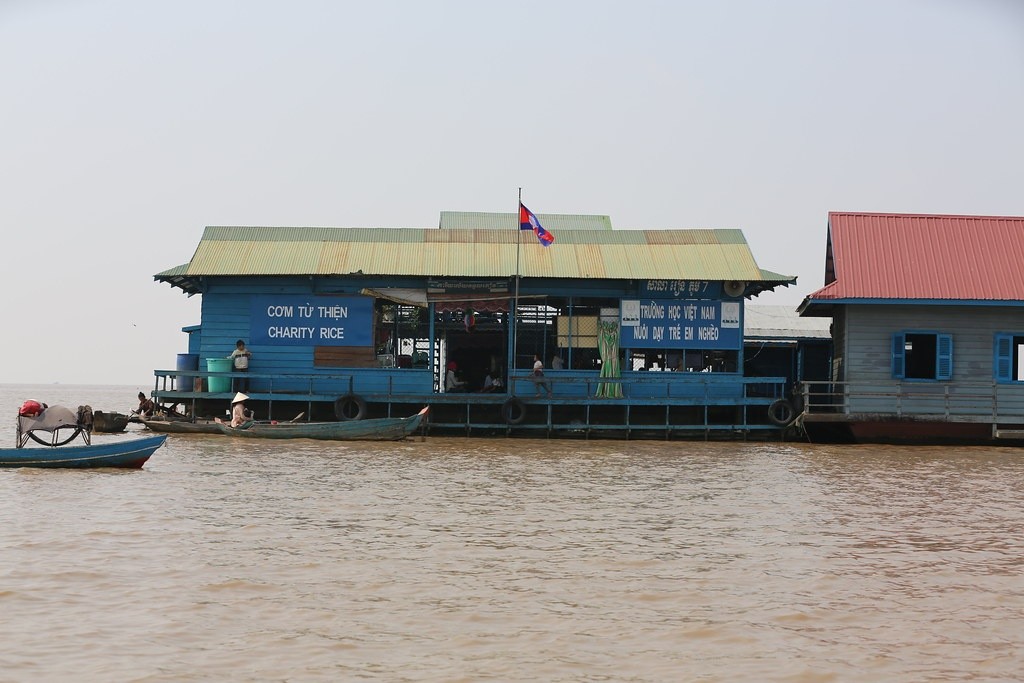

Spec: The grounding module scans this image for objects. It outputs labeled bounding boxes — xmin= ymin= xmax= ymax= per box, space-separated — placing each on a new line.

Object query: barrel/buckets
xmin=206 ymin=358 xmax=233 ymax=392
xmin=176 ymin=354 xmax=199 ymax=392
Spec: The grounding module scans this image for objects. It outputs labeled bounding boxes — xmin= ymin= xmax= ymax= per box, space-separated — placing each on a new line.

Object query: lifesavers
xmin=500 ymin=397 xmax=528 ymax=425
xmin=766 ymin=397 xmax=794 ymax=427
xmin=333 ymin=392 xmax=368 ymax=422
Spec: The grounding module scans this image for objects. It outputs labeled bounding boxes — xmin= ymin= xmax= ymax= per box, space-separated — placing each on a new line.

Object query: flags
xmin=519 ymin=203 xmax=554 ymax=246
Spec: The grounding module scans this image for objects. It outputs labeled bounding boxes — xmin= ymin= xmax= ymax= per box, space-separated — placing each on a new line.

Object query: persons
xmin=446 ymin=360 xmax=468 ymax=394
xmin=552 ymin=352 xmax=567 ymax=369
xmin=226 ymin=339 xmax=252 ymax=393
xmin=678 ymin=357 xmax=683 ymax=372
xmin=135 ymin=392 xmax=153 ymax=416
xmin=478 ymin=369 xmax=504 ymax=394
xmin=532 ymin=354 xmax=552 ymax=399
xmin=230 ymin=392 xmax=256 ymax=429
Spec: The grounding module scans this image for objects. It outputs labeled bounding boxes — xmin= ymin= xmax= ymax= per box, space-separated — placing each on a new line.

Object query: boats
xmin=0 ymin=400 xmax=169 ymax=468
xmin=77 ymin=405 xmax=128 ymax=434
xmin=129 ymin=416 xmax=150 ymax=423
xmin=144 ymin=420 xmax=230 ymax=434
xmin=149 ymin=210 xmax=832 ymax=442
xmin=215 ymin=406 xmax=429 ymax=441
xmin=796 ymin=210 xmax=1024 ymax=444
